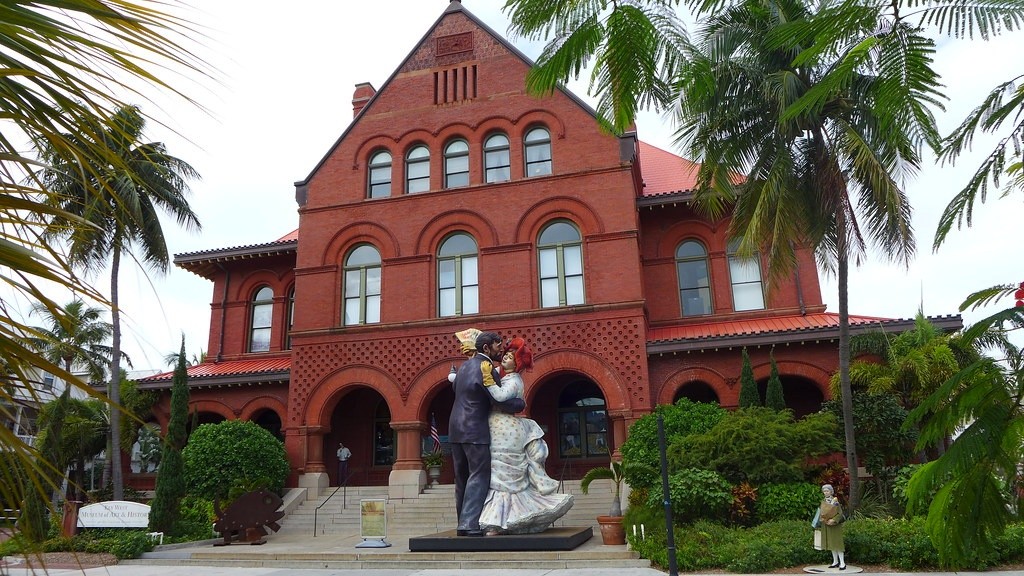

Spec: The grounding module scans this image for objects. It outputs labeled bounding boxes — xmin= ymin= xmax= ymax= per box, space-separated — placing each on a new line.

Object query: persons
xmin=336 ymin=441 xmax=352 ymax=486
xmin=819 ymin=484 xmax=846 ymax=571
xmin=447 ymin=331 xmax=527 ymax=537
xmin=460 ymin=337 xmax=575 ymax=536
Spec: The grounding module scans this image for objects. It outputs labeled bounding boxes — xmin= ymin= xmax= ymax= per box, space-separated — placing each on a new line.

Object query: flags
xmin=430 ymin=416 xmax=441 ymax=452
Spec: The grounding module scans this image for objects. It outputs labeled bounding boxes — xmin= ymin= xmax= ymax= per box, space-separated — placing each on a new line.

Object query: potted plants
xmin=422 ymin=448 xmax=448 ymax=485
xmin=229 ymin=475 xmax=272 ymax=541
xmin=129 ymin=423 xmax=157 ymax=490
xmin=579 ymin=456 xmax=659 ymax=545
